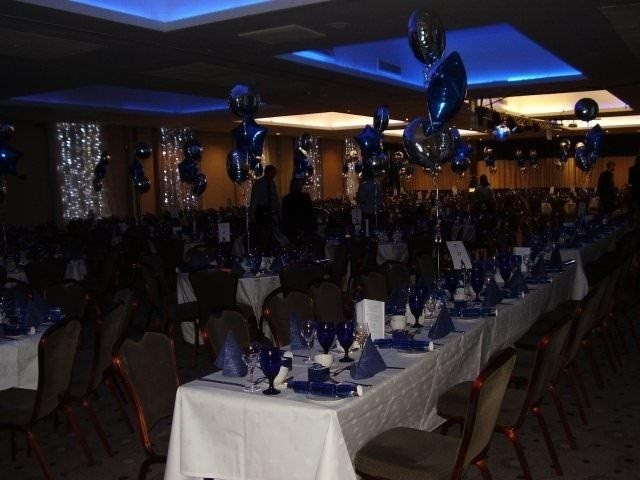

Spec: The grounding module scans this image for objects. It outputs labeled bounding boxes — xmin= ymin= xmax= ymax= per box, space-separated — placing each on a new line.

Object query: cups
xmin=313 ymin=353 xmax=333 ymax=369
xmin=273 ymin=366 xmax=289 ymax=385
xmin=308 ymin=367 xmax=329 ymax=382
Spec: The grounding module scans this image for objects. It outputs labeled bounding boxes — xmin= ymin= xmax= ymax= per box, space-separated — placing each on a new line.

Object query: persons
xmin=596 ymin=159 xmax=617 ymax=211
xmin=249 ymin=163 xmax=279 ymax=214
xmin=628 ymin=153 xmax=639 ymax=192
xmin=354 ymin=168 xmax=379 ymax=229
xmin=282 ymin=180 xmax=311 ymax=208
xmin=469 ymin=176 xmax=478 ymax=188
xmin=475 ymin=175 xmax=496 ymax=202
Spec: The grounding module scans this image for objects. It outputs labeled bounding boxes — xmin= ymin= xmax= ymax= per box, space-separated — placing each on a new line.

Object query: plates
xmin=266 ymin=382 xmax=290 ymax=389
xmin=306 ymin=394 xmax=344 ymax=403
xmin=394 ymin=348 xmax=428 ymax=356
xmin=4 ymin=321 xmax=55 ymax=341
xmin=329 ymin=363 xmax=339 ymax=371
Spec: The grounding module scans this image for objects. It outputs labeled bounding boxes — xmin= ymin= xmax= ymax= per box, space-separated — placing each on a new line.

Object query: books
xmin=93 ymin=152 xmax=111 ymax=193
xmin=223 ymin=85 xmax=267 ymax=187
xmin=0 ymin=119 xmax=22 ymax=173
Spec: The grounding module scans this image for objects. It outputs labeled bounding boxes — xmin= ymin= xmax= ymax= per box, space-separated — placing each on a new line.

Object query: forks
xmin=327 ymin=377 xmax=373 ymax=387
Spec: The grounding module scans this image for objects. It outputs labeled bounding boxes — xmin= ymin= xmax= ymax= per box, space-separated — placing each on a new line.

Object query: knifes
xmin=197 ymin=377 xmax=245 ymax=388
xmin=384 ymin=366 xmax=406 ymax=369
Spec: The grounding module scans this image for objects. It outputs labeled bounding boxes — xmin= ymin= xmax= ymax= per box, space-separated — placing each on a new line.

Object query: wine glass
xmin=336 ymin=321 xmax=355 ymax=362
xmin=388 ymin=240 xmax=560 ymax=332
xmin=317 ymin=321 xmax=335 ymax=353
xmin=298 ymin=321 xmax=317 ymax=363
xmin=240 ymin=342 xmax=261 ymax=393
xmin=259 ymin=346 xmax=282 ymax=395
xmin=354 ymin=323 xmax=370 ymax=351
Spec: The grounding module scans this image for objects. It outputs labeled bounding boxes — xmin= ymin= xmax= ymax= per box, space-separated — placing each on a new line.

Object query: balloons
xmin=128 ymin=142 xmax=154 ymax=197
xmin=177 ymin=137 xmax=209 ymax=199
xmin=571 ymin=96 xmax=608 ymax=173
xmin=403 ymin=3 xmax=472 ymax=174
xmin=295 ymin=103 xmax=412 ymax=184
xmin=479 ymin=114 xmax=570 ymax=179
